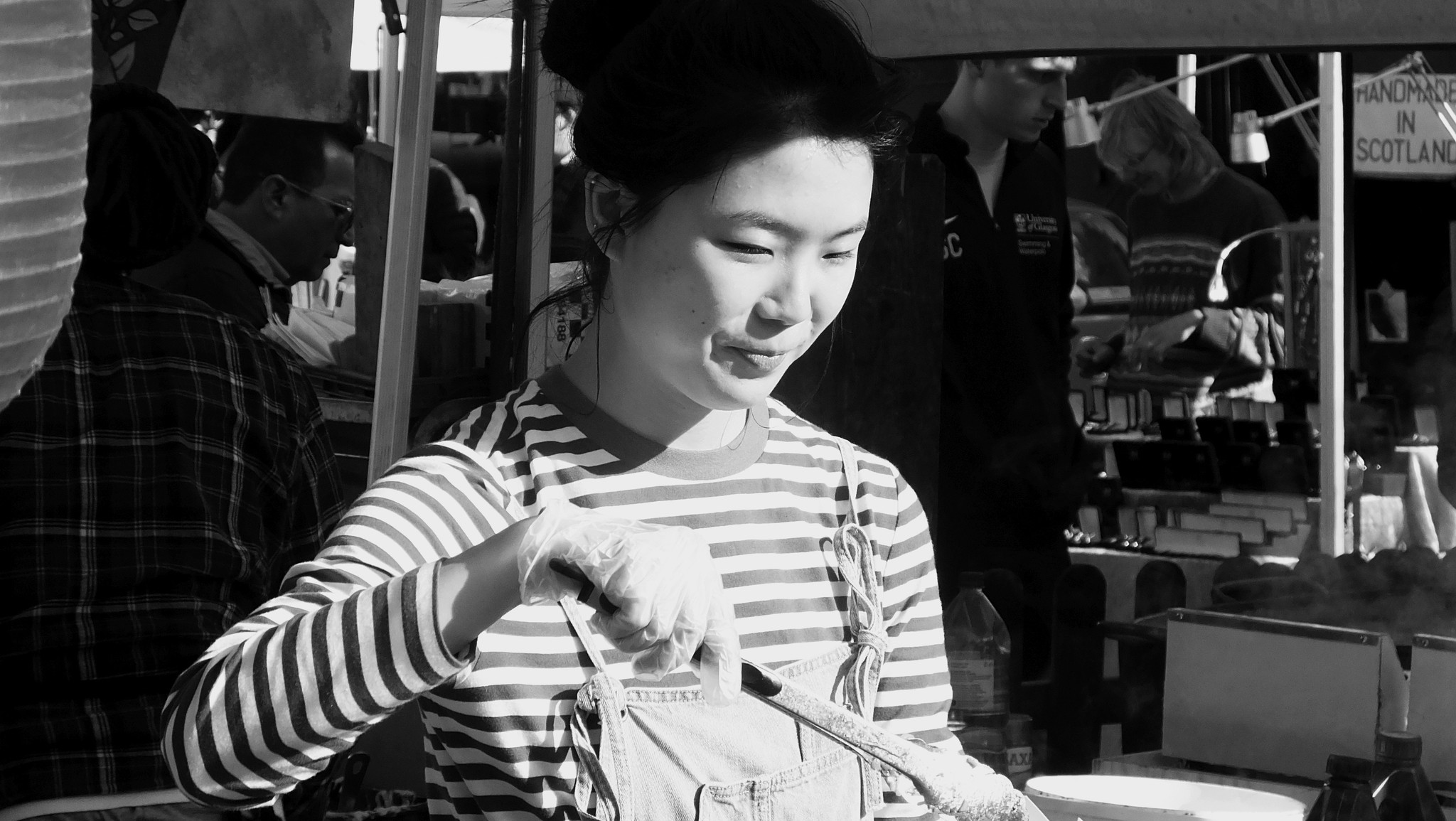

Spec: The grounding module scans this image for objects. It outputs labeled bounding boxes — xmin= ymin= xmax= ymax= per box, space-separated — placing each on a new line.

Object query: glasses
xmin=1120 ymin=136 xmax=1162 ymax=175
xmin=275 ymin=174 xmax=359 ymax=234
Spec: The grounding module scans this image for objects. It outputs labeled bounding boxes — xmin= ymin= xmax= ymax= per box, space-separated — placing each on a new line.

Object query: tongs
xmin=550 ymin=559 xmax=1021 ymax=821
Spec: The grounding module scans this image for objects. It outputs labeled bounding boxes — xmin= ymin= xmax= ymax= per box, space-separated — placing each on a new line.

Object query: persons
xmin=1077 ymin=77 xmax=1287 ymax=465
xmin=158 ymin=0 xmax=968 ymax=820
xmin=0 ymin=1 xmax=361 ymax=821
xmin=775 ymin=56 xmax=1090 ymax=779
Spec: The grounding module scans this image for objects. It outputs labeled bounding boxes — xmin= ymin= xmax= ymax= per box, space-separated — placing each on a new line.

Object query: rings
xmin=1147 ymin=342 xmax=1153 ymax=349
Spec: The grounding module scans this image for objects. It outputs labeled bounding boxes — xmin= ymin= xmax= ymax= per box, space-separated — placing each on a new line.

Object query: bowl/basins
xmin=1023 ymin=772 xmax=1306 ymax=821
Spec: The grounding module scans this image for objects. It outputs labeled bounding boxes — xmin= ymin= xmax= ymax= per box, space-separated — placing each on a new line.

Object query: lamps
xmin=1061 ymin=50 xmax=1319 ymax=161
xmin=1230 ymin=50 xmax=1456 ymax=141
xmin=1207 ymin=219 xmax=1319 ymax=303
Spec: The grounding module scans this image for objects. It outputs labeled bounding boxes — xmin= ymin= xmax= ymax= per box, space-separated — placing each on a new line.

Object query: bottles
xmin=1306 ymin=753 xmax=1379 ymax=821
xmin=1371 ymin=731 xmax=1447 ymax=821
